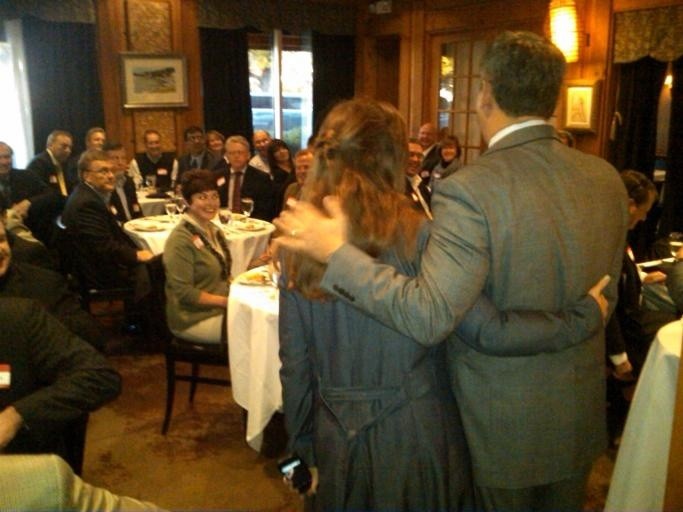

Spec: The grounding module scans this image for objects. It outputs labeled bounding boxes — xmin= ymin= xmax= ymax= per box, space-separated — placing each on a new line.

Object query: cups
xmin=669 ymin=233 xmax=683 ymax=257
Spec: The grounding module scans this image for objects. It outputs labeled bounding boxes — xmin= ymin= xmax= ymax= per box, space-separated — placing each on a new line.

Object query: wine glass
xmin=241 ymin=197 xmax=253 ymax=225
xmin=164 ymin=195 xmax=186 ymax=224
xmin=134 ymin=174 xmax=156 ymax=194
xmin=217 ymin=209 xmax=232 ymax=229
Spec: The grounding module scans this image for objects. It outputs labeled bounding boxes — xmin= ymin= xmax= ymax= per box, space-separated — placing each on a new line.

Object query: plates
xmin=662 ymin=257 xmax=676 ymax=264
xmin=231 ymin=214 xmax=265 ymax=231
xmin=133 ymin=220 xmax=166 ymax=232
xmin=237 ymin=272 xmax=273 ymax=286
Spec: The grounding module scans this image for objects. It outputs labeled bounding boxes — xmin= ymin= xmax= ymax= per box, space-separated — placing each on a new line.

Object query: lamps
xmin=547 ymin=0 xmax=590 ymax=69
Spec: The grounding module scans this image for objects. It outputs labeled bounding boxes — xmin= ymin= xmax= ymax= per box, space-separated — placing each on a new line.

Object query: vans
xmin=250 ymin=94 xmax=303 ymax=136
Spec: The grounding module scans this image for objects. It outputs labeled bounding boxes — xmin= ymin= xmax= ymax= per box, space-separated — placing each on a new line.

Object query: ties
xmin=231 ymin=171 xmax=243 ymax=215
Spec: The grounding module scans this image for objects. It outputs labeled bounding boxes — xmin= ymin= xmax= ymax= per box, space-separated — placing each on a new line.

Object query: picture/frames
xmin=118 ymin=52 xmax=191 ymax=112
xmin=555 ymin=81 xmax=601 ymax=137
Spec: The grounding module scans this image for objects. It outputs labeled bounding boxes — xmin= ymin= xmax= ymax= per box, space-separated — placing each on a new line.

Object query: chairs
xmin=55 ymin=214 xmax=140 ymax=356
xmin=147 ymin=251 xmax=248 ymax=439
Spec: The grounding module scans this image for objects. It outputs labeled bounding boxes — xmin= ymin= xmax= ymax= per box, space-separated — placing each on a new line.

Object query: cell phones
xmin=278 ymin=455 xmax=320 ymax=496
